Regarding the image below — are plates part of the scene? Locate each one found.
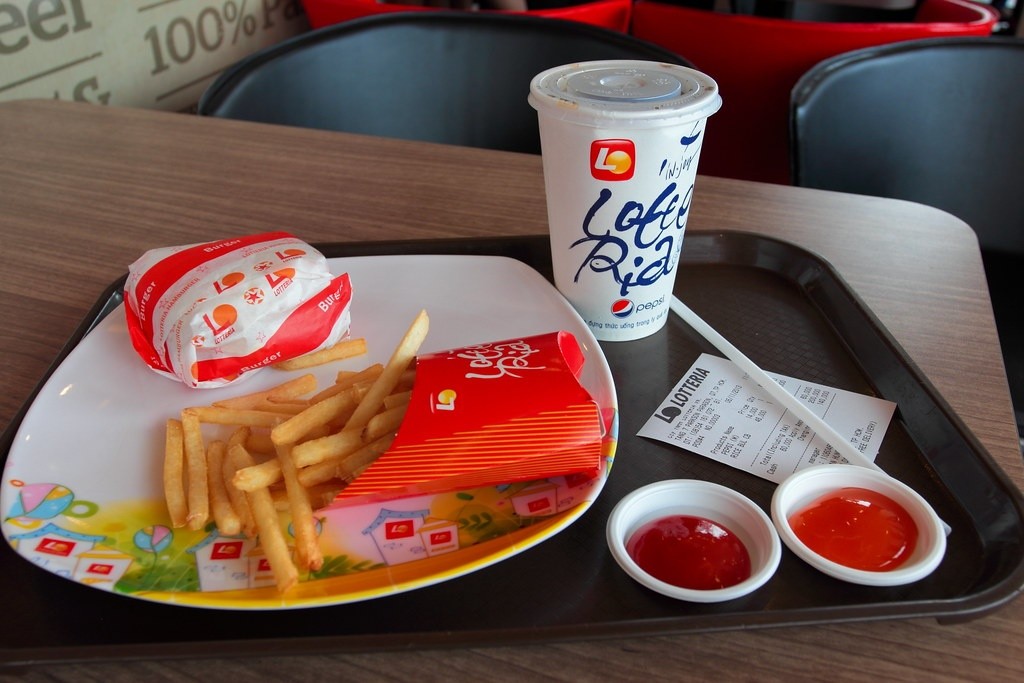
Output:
[0,255,619,609]
[769,463,948,586]
[606,477,781,602]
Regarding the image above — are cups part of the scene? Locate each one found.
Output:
[526,60,721,342]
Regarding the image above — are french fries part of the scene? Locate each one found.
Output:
[164,309,430,595]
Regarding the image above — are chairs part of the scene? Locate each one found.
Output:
[192,0,1024,255]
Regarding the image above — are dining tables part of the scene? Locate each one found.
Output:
[0,98,1024,683]
